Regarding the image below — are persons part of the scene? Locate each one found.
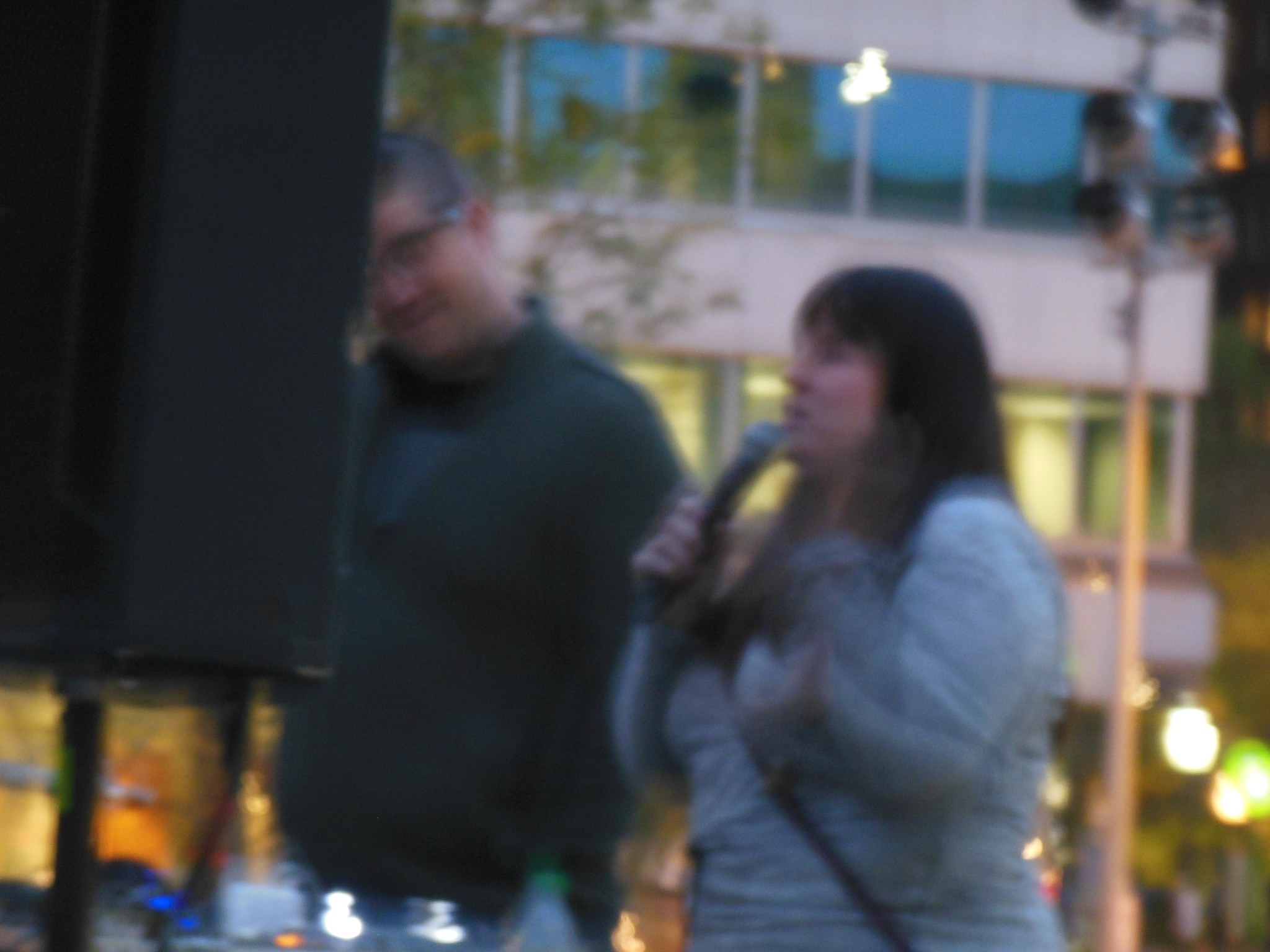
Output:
[271,132,696,952]
[609,266,1074,952]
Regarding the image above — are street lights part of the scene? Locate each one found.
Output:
[1062,1,1245,952]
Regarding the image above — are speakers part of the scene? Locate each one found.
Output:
[0,1,393,686]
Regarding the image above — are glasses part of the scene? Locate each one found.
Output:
[362,206,463,283]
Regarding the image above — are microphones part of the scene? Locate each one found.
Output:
[703,424,788,537]
